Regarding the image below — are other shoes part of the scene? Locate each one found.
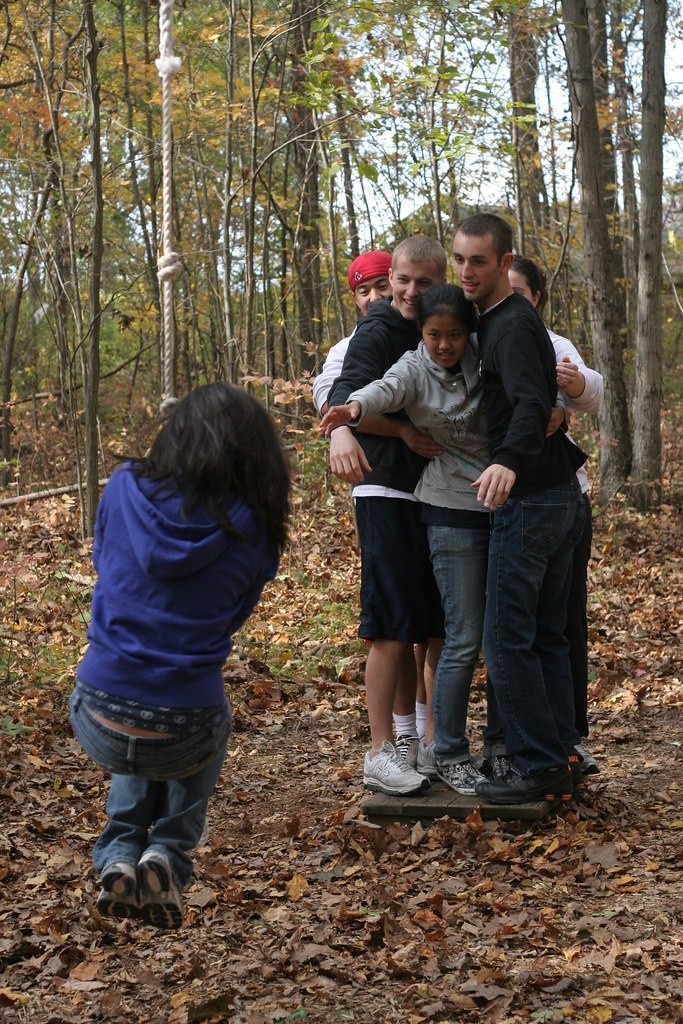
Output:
[136,852,186,930]
[97,863,140,918]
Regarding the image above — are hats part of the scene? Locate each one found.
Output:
[347,251,393,293]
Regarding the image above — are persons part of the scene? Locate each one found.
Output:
[313,213,604,806]
[69,382,291,930]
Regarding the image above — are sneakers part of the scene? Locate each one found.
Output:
[475,765,574,803]
[576,741,600,776]
[489,754,510,780]
[568,763,581,781]
[417,741,489,775]
[393,734,419,767]
[362,741,429,796]
[437,758,490,797]
